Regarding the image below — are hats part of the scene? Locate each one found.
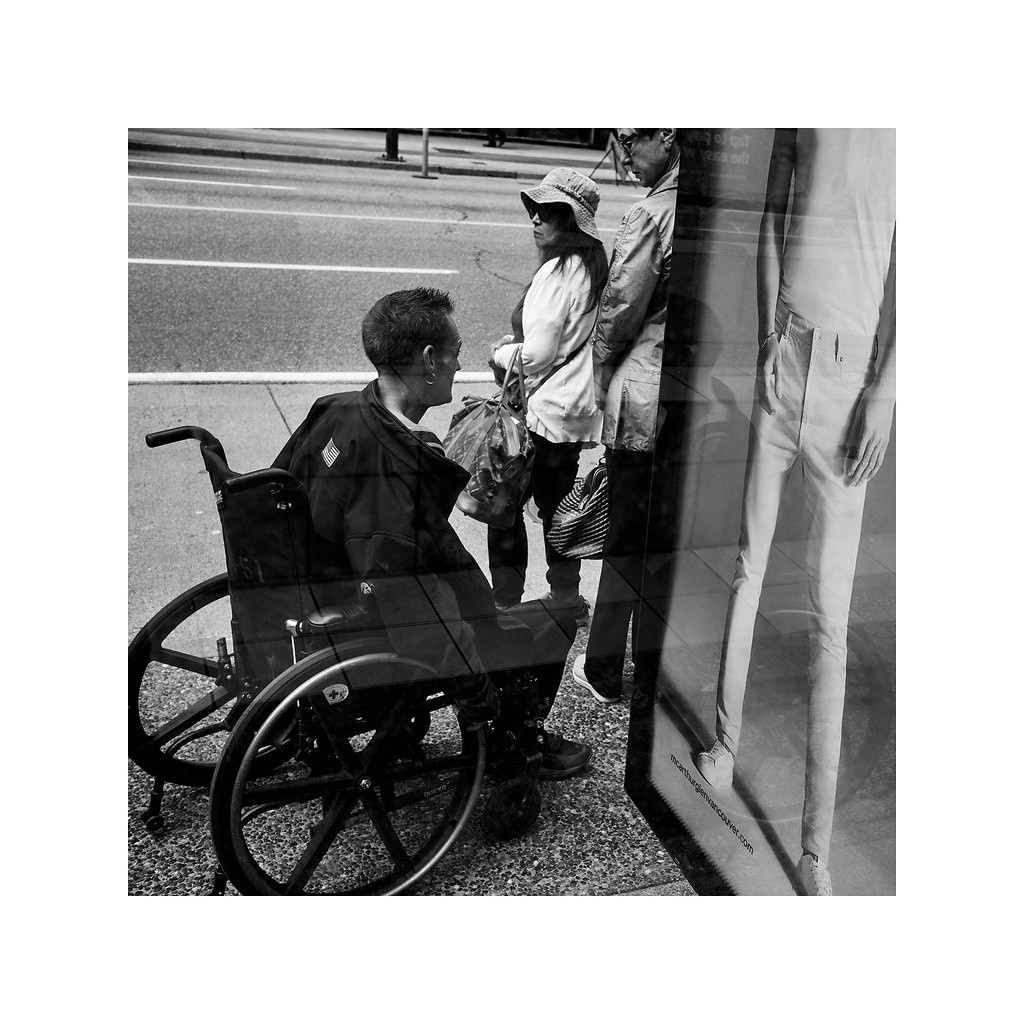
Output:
[519,168,601,241]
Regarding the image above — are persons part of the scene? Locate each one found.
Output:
[696,128,896,895]
[573,129,682,705]
[270,287,589,782]
[480,163,609,629]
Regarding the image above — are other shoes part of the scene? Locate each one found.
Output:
[483,717,592,778]
[483,135,506,147]
[542,591,589,626]
[572,653,619,703]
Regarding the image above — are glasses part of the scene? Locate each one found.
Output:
[618,133,636,152]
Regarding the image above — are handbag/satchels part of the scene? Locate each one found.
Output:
[443,344,535,531]
[546,456,610,560]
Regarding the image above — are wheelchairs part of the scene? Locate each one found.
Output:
[129,424,560,897]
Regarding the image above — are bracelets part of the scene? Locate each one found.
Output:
[758,330,777,353]
